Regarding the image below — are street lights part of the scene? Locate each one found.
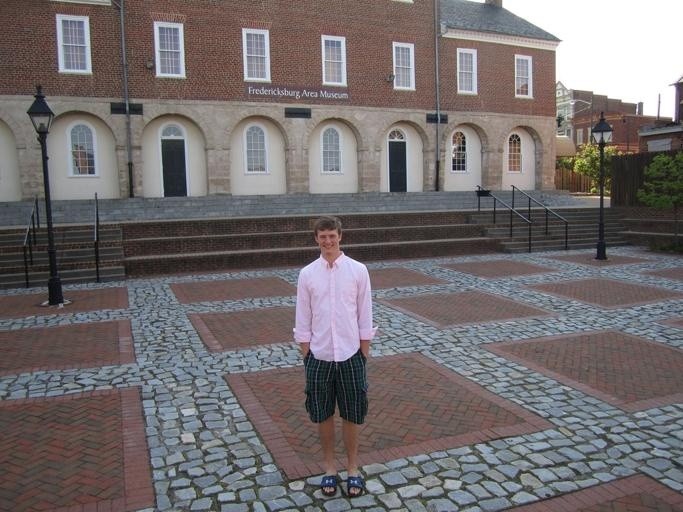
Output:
[569,97,594,146]
[24,82,65,308]
[591,109,616,261]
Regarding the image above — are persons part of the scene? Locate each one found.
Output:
[293,214,378,498]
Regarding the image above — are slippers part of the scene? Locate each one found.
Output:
[320,475,337,497]
[347,476,363,497]
